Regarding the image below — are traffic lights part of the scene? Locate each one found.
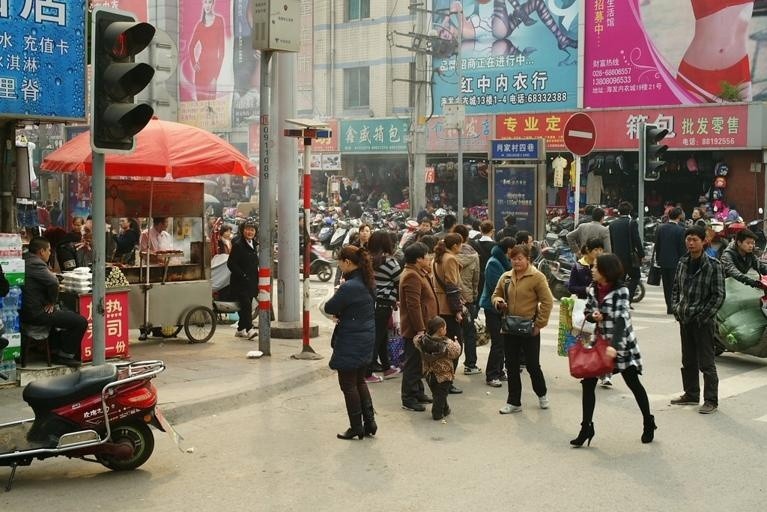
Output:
[94,10,155,150]
[645,125,668,179]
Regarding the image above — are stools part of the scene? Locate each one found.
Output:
[21,323,52,368]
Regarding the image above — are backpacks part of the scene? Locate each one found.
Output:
[593,153,698,175]
[436,162,488,178]
[712,162,729,213]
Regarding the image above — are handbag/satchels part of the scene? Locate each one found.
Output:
[648,266,661,285]
[110,249,135,265]
[459,324,490,346]
[387,336,401,367]
[375,297,395,316]
[567,337,616,378]
[571,299,597,333]
[500,314,534,337]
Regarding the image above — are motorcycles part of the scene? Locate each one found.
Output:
[0,327,184,492]
[220,200,457,283]
[713,254,767,358]
[538,204,767,304]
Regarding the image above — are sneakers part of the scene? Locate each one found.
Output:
[599,378,613,387]
[235,329,258,340]
[698,400,717,413]
[53,355,81,367]
[670,392,698,404]
[337,365,549,440]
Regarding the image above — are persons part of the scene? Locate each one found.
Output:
[570,252,657,446]
[187,0,227,100]
[669,225,726,413]
[227,221,258,340]
[432,0,579,56]
[324,203,553,440]
[720,229,767,288]
[578,205,595,226]
[451,38,536,63]
[609,202,645,309]
[675,0,754,103]
[339,178,410,218]
[216,224,232,254]
[568,237,613,386]
[654,201,708,314]
[0,213,176,355]
[566,207,612,254]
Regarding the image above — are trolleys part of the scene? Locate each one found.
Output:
[106,241,217,344]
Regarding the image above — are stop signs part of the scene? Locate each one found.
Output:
[564,113,597,156]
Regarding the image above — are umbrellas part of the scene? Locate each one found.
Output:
[40,116,259,327]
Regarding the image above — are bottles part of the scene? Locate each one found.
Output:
[0,232,23,260]
[18,201,39,226]
[0,358,17,383]
[0,286,23,335]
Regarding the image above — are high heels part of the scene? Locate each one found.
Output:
[641,414,657,443]
[570,422,594,447]
